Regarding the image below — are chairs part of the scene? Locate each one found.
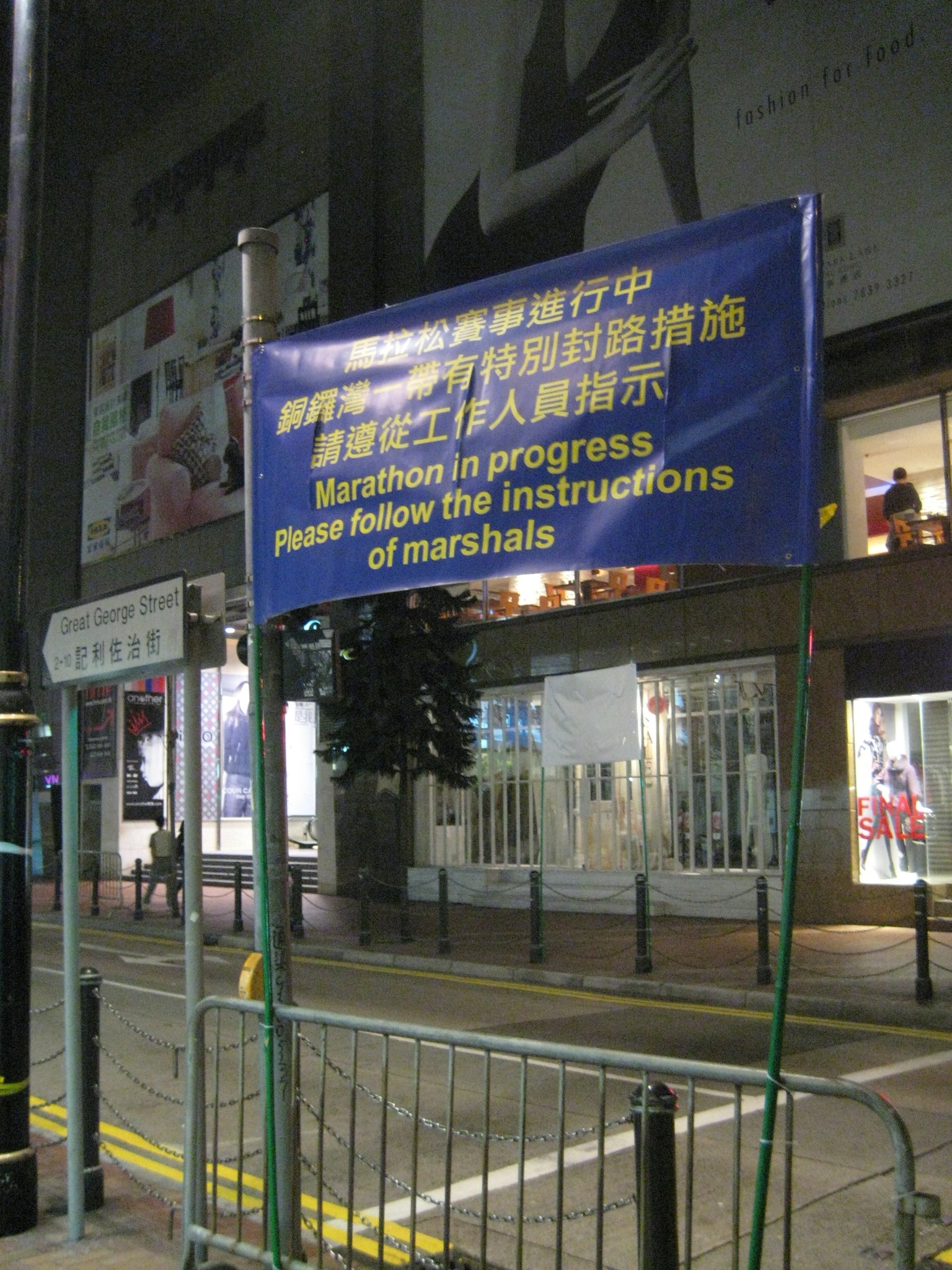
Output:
[892,517,919,548]
[466,570,667,620]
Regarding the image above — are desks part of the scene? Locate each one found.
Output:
[554,579,609,602]
[907,516,951,544]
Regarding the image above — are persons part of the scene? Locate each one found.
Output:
[424,0,703,299]
[882,467,922,552]
[857,703,897,878]
[127,709,164,800]
[141,816,184,918]
[873,741,932,873]
[221,681,252,817]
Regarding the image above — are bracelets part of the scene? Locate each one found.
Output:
[570,141,580,183]
